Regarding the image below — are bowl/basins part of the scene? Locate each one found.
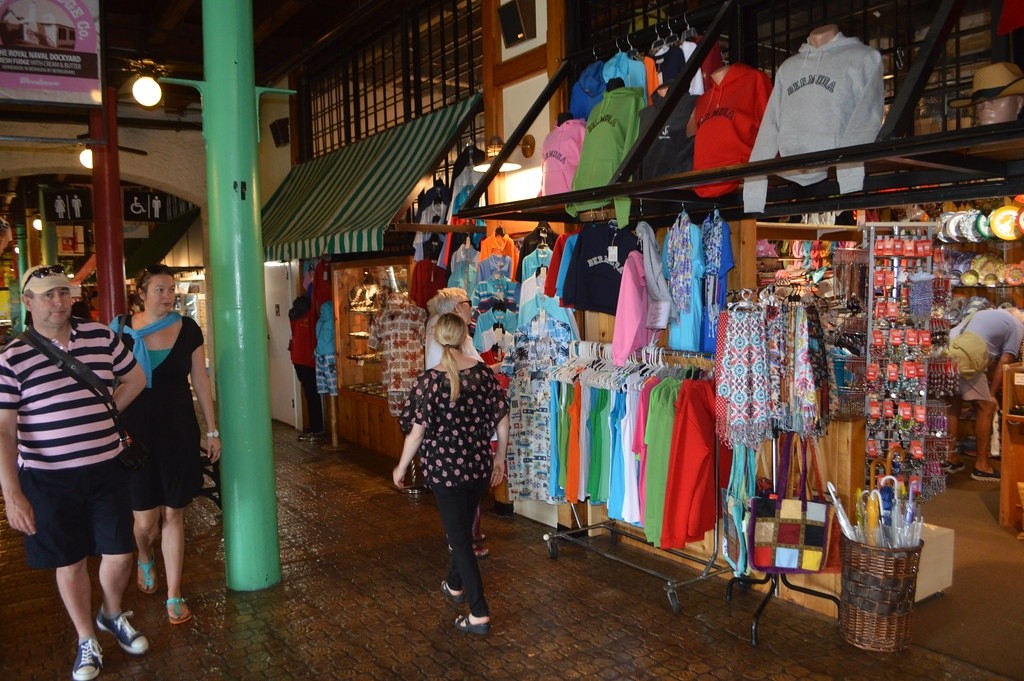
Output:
[984,273,997,286]
[961,270,979,286]
[946,270,962,284]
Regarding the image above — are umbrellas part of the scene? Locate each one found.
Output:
[827,446,924,547]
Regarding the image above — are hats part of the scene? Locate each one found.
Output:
[22,265,72,293]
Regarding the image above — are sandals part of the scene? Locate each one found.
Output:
[470,532,486,540]
[448,540,489,558]
[167,598,191,625]
[137,552,158,594]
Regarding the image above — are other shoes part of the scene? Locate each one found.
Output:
[945,461,964,474]
[971,467,1001,482]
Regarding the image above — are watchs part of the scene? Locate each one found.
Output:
[207,429,219,438]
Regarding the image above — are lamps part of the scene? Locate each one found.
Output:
[78,138,93,171]
[124,60,167,107]
[474,135,535,174]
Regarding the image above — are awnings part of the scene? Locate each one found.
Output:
[261,91,482,260]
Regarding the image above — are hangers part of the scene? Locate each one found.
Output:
[407,172,448,270]
[577,15,709,69]
[521,221,558,333]
[720,282,828,319]
[548,341,716,401]
[448,166,514,362]
[454,137,484,163]
[556,199,730,270]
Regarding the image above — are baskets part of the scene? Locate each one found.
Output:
[838,527,925,652]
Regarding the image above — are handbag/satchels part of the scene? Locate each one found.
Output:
[938,331,990,380]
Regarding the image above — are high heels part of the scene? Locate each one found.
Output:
[440,579,465,602]
[454,616,491,635]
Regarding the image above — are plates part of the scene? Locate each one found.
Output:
[936,206,1024,243]
[951,251,1024,286]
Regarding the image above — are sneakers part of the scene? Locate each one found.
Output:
[96,607,149,655]
[72,636,102,681]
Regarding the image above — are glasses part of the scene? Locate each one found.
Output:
[22,265,68,295]
[453,300,472,310]
[138,264,170,289]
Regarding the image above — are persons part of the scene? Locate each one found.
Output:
[392,312,510,632]
[425,287,490,557]
[693,63,773,208]
[368,292,428,416]
[289,295,323,441]
[0,264,148,681]
[949,62,1024,125]
[949,306,1024,482]
[542,78,646,228]
[742,23,884,215]
[72,301,92,318]
[108,263,221,624]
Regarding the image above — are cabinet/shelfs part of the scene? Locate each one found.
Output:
[947,240,1024,289]
[1000,361,1023,530]
[330,256,415,458]
